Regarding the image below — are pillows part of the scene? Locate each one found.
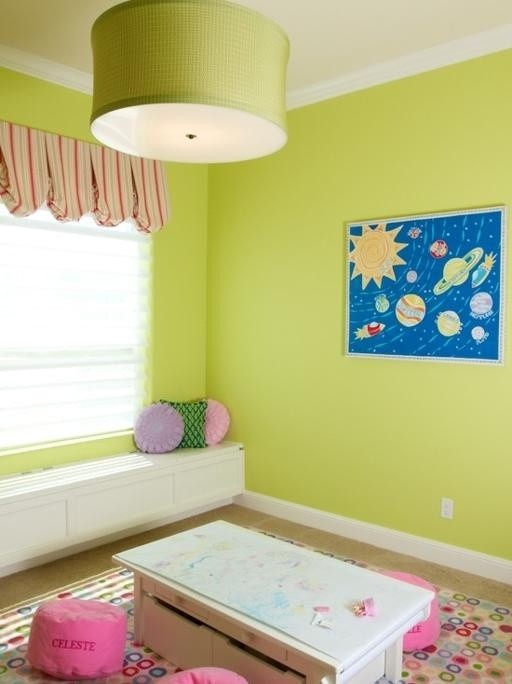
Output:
[134,403,185,454]
[159,399,209,447]
[207,399,232,447]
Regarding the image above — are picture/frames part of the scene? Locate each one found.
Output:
[341,205,505,366]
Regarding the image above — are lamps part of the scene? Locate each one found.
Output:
[90,1,290,165]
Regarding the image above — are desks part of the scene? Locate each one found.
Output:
[111,521,435,684]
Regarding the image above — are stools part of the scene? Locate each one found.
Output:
[384,572,440,652]
[25,599,128,679]
[153,667,247,684]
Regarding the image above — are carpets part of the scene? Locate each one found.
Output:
[0,528,512,684]
